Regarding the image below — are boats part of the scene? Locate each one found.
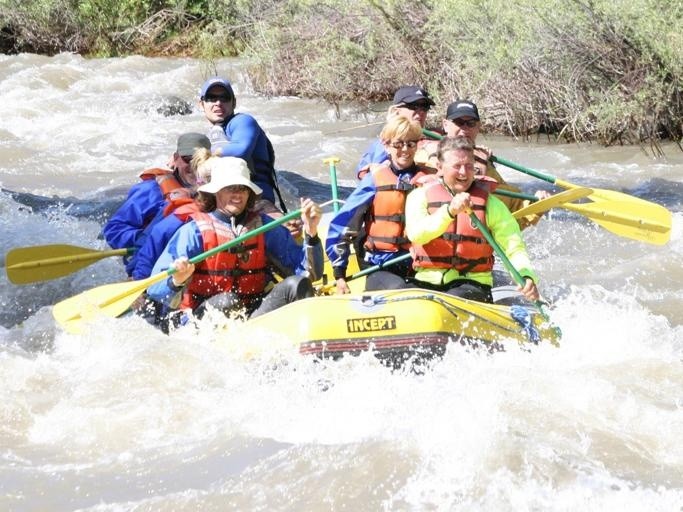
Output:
[238,211,562,371]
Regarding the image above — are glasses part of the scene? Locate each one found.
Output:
[180,156,191,163]
[452,118,475,126]
[392,140,416,149]
[226,185,247,192]
[204,94,230,102]
[401,103,429,111]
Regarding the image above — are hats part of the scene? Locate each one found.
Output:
[176,133,210,156]
[446,100,478,120]
[200,76,233,99]
[196,157,263,197]
[393,86,435,105]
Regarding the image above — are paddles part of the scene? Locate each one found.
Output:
[319,186,591,291]
[493,188,672,245]
[6,245,139,283]
[50,209,301,333]
[419,128,669,212]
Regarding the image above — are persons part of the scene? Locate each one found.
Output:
[144,155,324,331]
[196,76,279,203]
[357,85,492,184]
[406,135,539,307]
[415,100,551,234]
[100,129,301,311]
[326,117,432,298]
